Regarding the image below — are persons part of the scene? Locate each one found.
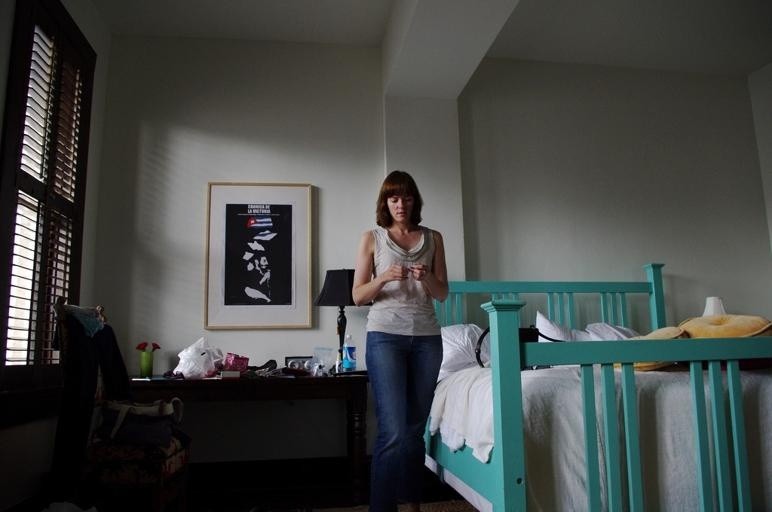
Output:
[352,170,449,512]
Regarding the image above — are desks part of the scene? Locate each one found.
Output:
[128,372,368,505]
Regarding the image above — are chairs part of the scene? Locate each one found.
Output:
[54,297,193,512]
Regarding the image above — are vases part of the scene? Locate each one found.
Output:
[141,352,154,378]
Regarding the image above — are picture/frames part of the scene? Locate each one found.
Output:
[285,356,314,373]
[203,181,313,329]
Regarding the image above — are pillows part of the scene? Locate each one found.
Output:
[678,313,772,366]
[436,323,491,383]
[535,309,640,367]
[611,325,690,371]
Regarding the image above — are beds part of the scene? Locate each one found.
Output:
[421,263,772,512]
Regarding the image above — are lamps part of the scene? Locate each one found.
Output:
[314,268,375,375]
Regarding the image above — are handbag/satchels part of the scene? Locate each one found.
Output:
[104,396,183,444]
[514,324,541,344]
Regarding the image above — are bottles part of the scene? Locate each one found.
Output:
[343,335,356,372]
[703,297,728,316]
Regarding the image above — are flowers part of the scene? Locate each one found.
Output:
[135,341,160,352]
